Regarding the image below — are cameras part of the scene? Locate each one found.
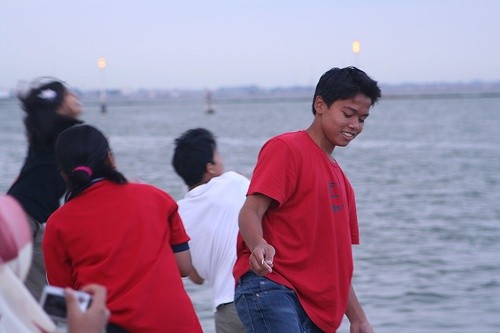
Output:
[40,285,93,324]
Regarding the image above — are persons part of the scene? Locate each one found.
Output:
[0,79,204,333]
[232,66,382,333]
[171,128,251,333]
